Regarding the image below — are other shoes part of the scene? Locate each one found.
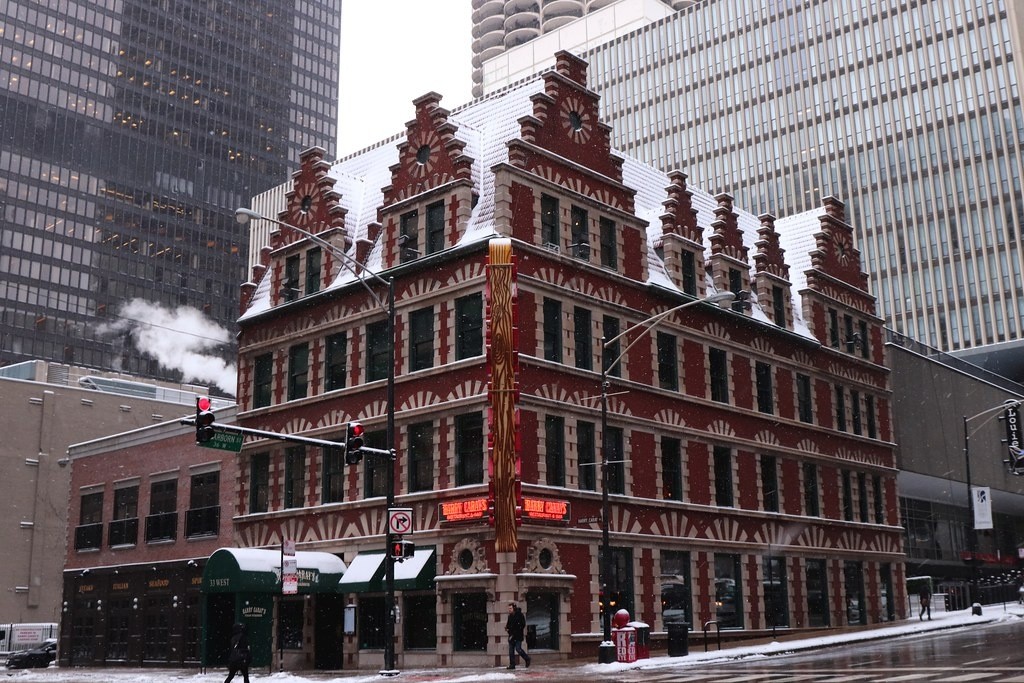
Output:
[507,664,516,670]
[919,614,922,621]
[928,616,931,620]
[525,657,531,668]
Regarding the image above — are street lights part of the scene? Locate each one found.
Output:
[594,288,737,669]
[233,208,401,681]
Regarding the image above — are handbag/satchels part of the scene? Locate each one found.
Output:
[228,633,250,668]
[921,599,930,607]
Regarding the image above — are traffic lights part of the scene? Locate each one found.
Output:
[195,396,215,443]
[343,421,364,467]
[390,540,414,558]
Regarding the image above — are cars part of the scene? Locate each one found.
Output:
[4,638,58,669]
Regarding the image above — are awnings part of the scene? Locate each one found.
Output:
[202,547,347,597]
[338,549,435,591]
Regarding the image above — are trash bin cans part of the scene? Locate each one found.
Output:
[667,621,690,657]
[598,640,616,665]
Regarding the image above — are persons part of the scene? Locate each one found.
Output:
[222,622,251,683]
[1019,583,1024,604]
[918,580,932,620]
[504,603,531,669]
